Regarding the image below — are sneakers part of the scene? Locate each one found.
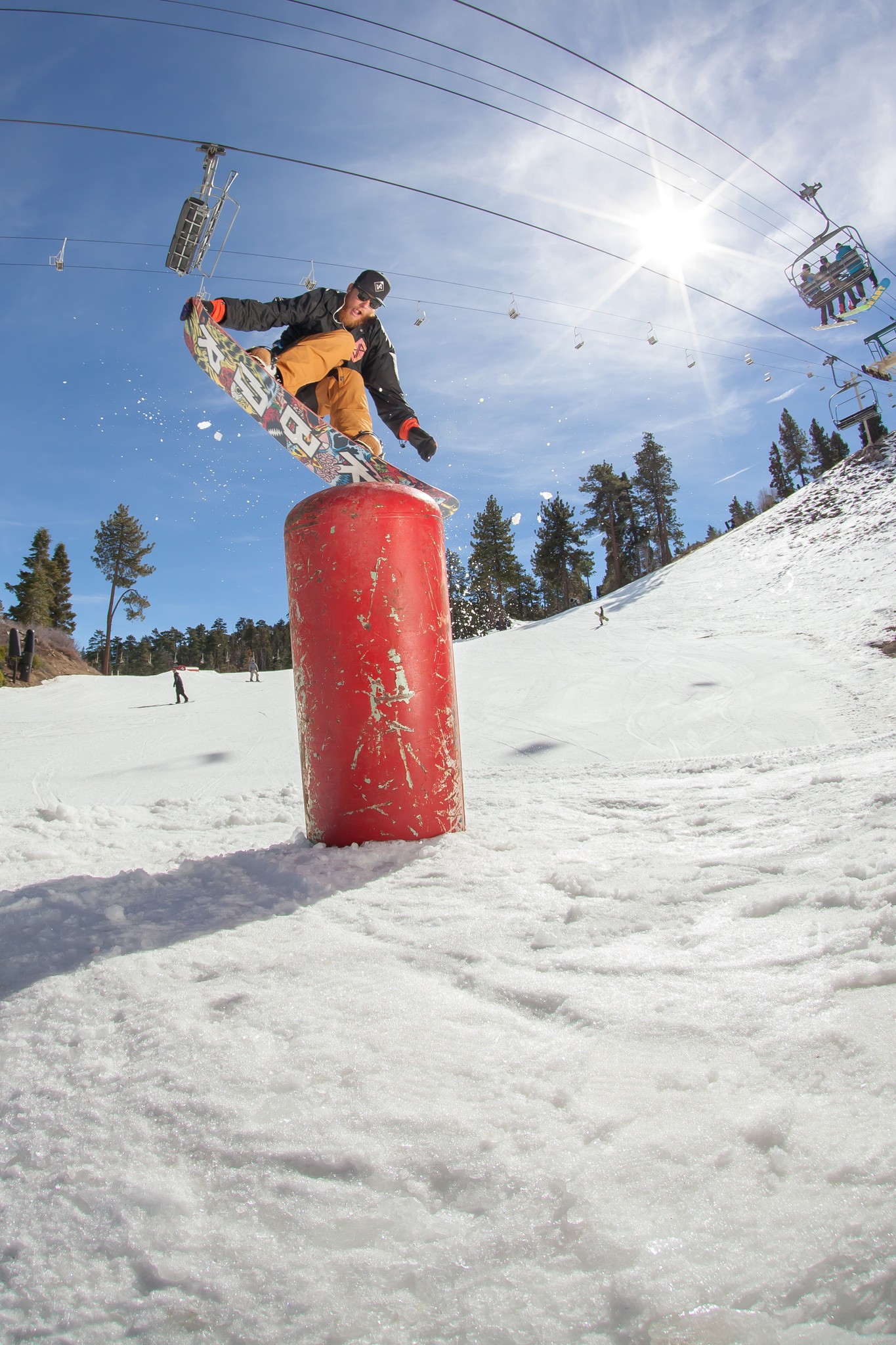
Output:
[820,280,880,326]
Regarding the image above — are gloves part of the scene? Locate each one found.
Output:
[180,297,226,323]
[398,418,437,463]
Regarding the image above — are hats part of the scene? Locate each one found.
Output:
[355,270,392,308]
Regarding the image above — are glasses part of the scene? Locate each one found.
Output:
[354,284,380,310]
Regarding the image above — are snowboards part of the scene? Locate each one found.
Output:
[595,611,609,622]
[836,305,868,319]
[860,278,890,311]
[245,680,264,682]
[169,700,195,705]
[811,319,858,331]
[184,296,461,521]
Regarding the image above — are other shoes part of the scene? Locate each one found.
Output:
[246,346,277,380]
[352,430,386,460]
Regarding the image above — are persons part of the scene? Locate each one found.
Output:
[172,668,188,704]
[800,264,838,326]
[179,269,437,463]
[820,256,861,315]
[599,606,603,625]
[249,660,260,682]
[835,243,879,303]
